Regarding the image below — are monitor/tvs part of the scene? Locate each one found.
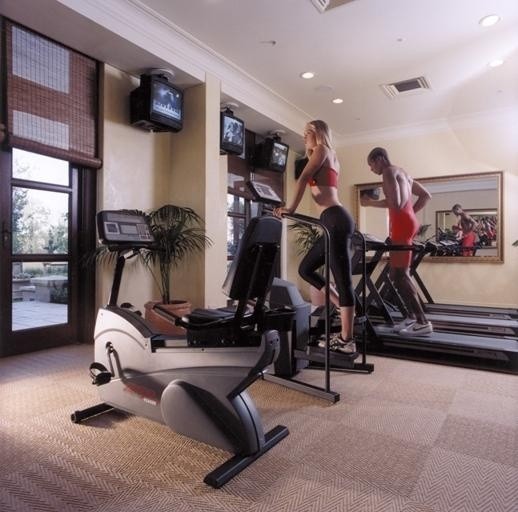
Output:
[360,188,381,200]
[295,157,309,179]
[130,75,183,132]
[220,111,244,155]
[256,140,289,173]
[247,180,282,205]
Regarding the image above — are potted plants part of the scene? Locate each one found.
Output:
[282,219,329,309]
[81,200,214,340]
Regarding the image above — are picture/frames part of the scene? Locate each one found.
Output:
[352,169,505,267]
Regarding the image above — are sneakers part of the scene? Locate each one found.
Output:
[399,320,433,338]
[393,317,417,334]
[318,333,357,354]
[335,307,357,320]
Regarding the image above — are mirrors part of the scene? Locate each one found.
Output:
[433,206,499,249]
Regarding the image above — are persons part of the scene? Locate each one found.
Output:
[272,121,358,355]
[358,146,434,338]
[167,92,180,112]
[447,202,499,256]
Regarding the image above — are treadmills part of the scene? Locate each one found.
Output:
[323,234,517,376]
